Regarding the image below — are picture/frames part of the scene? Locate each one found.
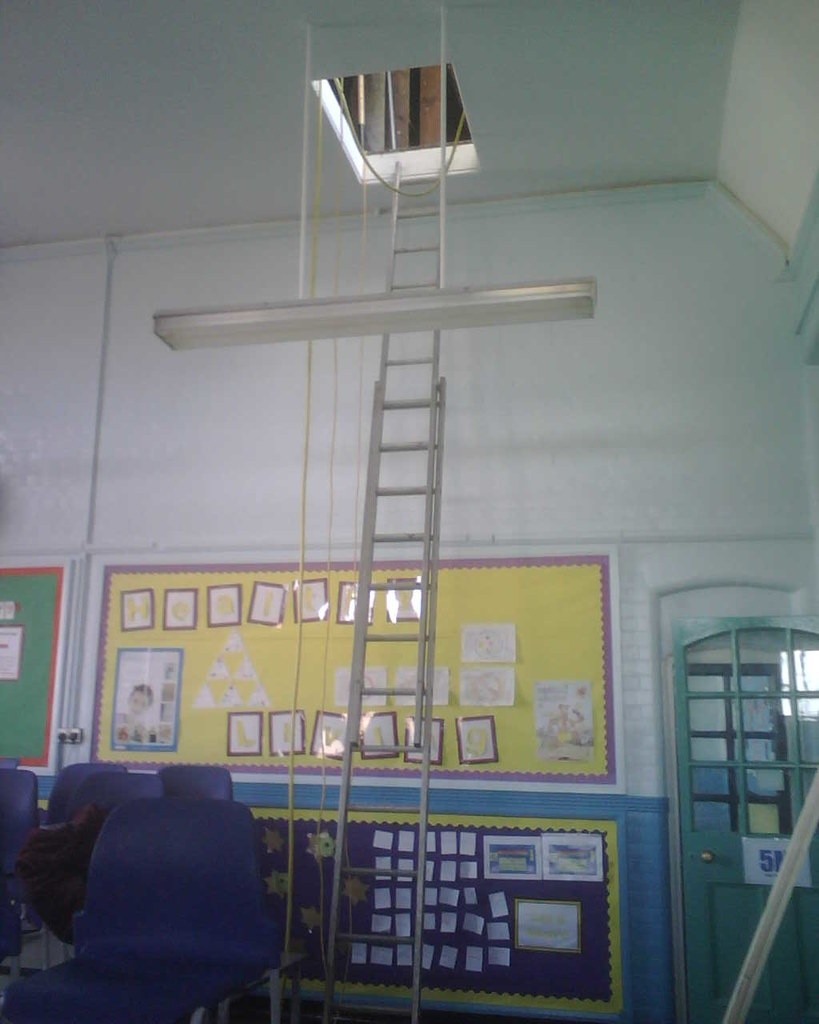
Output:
[514,898,582,955]
[119,588,155,632]
[109,646,184,752]
[292,577,329,624]
[246,580,288,629]
[335,581,375,625]
[207,583,242,628]
[226,711,263,757]
[162,588,198,631]
[0,624,26,682]
[357,711,400,759]
[309,709,348,762]
[267,709,306,757]
[386,575,422,623]
[403,716,444,766]
[455,715,498,765]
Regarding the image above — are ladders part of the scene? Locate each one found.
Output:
[320,148,447,1024]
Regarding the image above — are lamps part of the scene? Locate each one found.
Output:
[150,3,601,354]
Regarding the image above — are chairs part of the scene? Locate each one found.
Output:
[0,756,310,1024]
